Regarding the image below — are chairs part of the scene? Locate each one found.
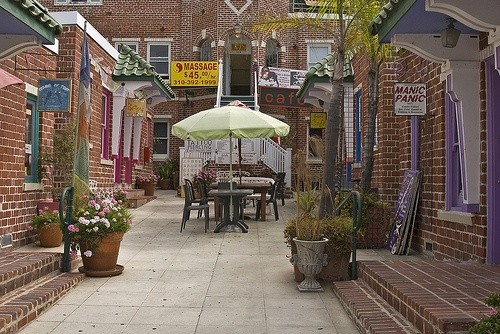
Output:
[180,172,286,233]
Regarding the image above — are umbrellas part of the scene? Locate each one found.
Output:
[171,102,290,233]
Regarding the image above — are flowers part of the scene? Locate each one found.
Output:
[26,206,67,236]
[137,171,160,183]
[67,188,136,258]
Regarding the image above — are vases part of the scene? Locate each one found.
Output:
[138,183,155,196]
[39,223,63,247]
[80,230,125,271]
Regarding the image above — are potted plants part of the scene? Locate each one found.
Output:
[283,135,393,292]
[159,160,178,189]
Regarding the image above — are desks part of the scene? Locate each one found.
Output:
[230,177,275,185]
[207,189,254,233]
[209,183,271,222]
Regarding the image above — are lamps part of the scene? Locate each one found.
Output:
[441,17,461,48]
[318,99,324,107]
[146,97,152,103]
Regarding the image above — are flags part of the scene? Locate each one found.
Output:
[71,20,92,216]
